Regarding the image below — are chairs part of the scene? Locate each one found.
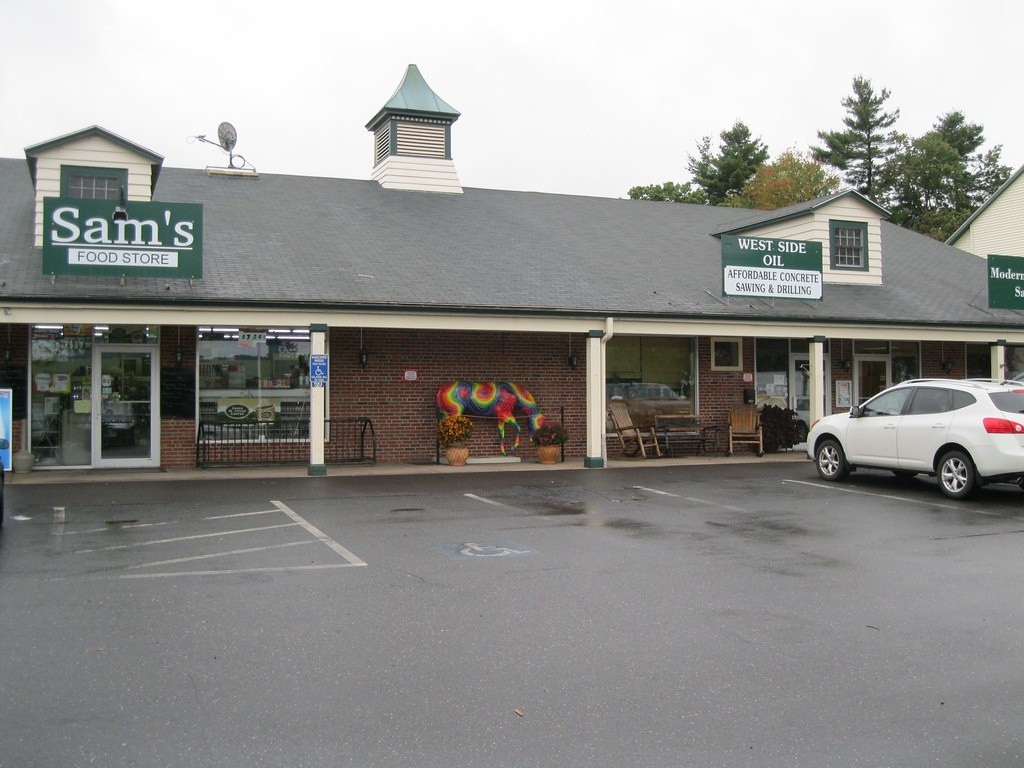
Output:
[726,405,763,456]
[608,397,663,462]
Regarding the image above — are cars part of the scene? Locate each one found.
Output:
[79,393,150,452]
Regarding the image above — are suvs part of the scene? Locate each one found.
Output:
[606,383,694,425]
[806,378,1024,501]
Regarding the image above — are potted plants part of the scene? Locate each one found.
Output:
[758,404,805,453]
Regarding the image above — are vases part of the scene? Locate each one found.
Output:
[537,445,561,464]
[446,444,468,466]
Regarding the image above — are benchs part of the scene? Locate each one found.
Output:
[653,414,721,458]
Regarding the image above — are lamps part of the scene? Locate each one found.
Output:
[942,355,953,374]
[840,355,852,373]
[111,184,128,222]
[359,347,368,368]
[568,349,578,370]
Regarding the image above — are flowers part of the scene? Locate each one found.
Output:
[530,424,568,448]
[438,415,474,447]
